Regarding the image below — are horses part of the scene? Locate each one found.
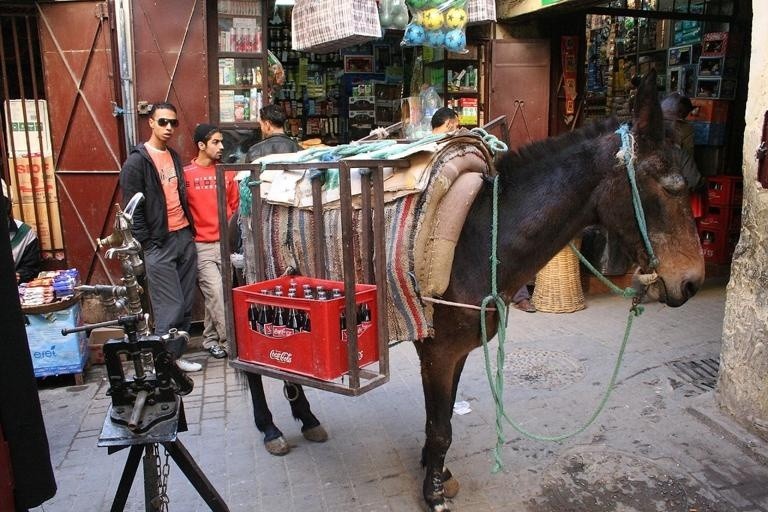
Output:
[226,68,704,512]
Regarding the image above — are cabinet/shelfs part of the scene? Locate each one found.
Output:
[423,31,482,134]
[208,2,271,131]
[271,1,422,146]
[584,12,670,126]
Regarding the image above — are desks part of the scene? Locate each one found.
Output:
[21,291,91,386]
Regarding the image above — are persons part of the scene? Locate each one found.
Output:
[120,101,205,373]
[657,139,705,192]
[245,104,306,163]
[660,93,695,157]
[0,177,44,287]
[430,107,461,134]
[182,123,240,360]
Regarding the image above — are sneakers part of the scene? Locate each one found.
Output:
[173,357,203,373]
[208,344,226,359]
[517,298,537,312]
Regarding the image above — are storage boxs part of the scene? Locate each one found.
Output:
[4,100,64,260]
[665,30,743,265]
[232,275,379,382]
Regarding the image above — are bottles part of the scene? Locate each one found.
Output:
[248,281,372,343]
[235,88,262,122]
[219,66,262,85]
[218,25,263,52]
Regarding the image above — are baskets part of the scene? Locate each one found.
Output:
[529,239,586,313]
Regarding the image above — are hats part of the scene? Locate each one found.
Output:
[192,123,221,145]
[661,94,700,119]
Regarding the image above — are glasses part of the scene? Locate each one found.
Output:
[154,118,180,129]
